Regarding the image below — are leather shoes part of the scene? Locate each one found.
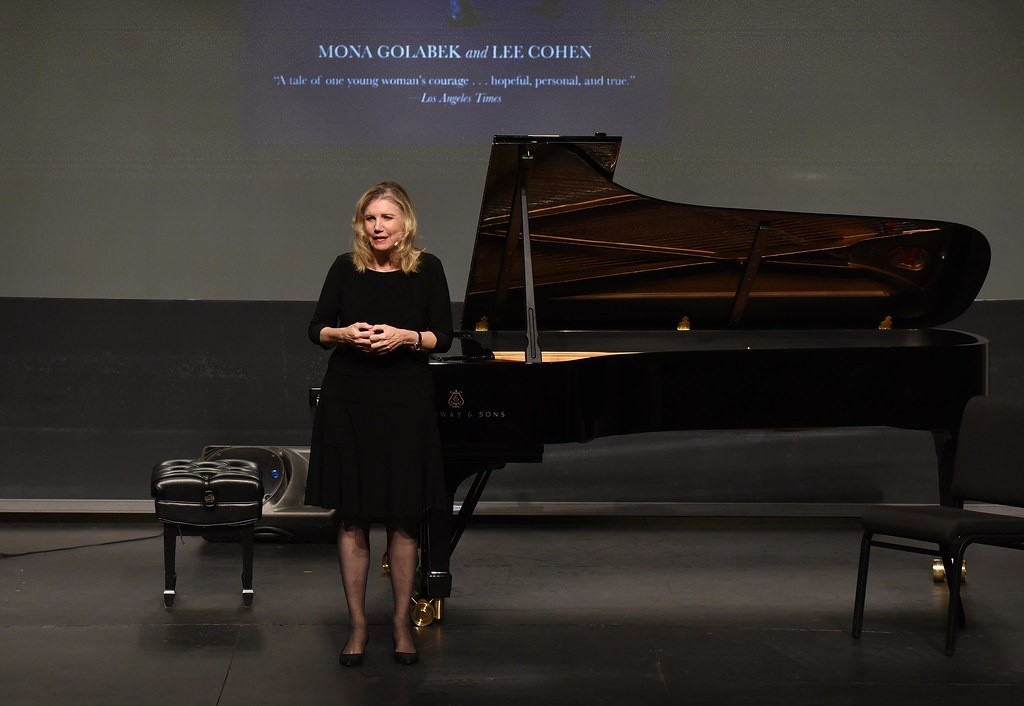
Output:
[339,634,370,667]
[390,634,419,665]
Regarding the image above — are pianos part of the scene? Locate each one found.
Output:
[377,130,995,629]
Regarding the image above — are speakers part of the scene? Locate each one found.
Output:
[203,445,334,539]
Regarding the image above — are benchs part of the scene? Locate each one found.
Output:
[150,459,265,607]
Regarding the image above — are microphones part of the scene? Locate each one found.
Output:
[394,239,401,246]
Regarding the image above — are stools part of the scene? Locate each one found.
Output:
[853,505,1024,658]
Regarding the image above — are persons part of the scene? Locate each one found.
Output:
[305,182,453,666]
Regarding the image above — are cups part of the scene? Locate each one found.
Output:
[462,331,492,359]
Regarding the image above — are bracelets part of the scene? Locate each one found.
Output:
[410,331,422,351]
